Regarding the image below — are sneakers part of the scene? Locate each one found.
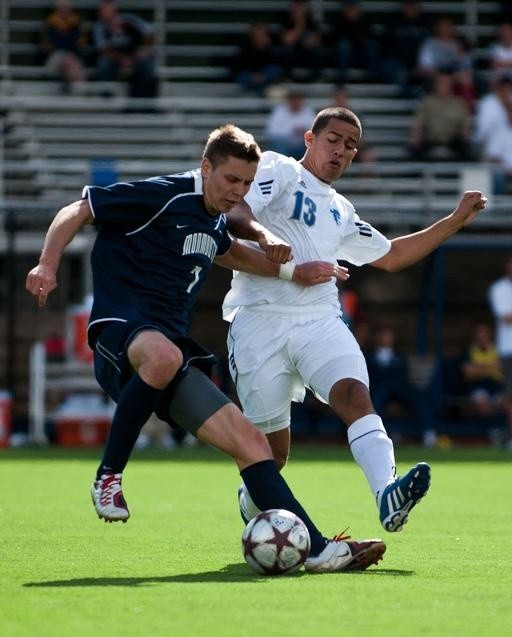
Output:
[375,461,431,532]
[238,487,255,524]
[91,472,129,523]
[304,537,386,572]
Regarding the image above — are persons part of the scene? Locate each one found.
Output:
[26,122,388,574]
[227,1,512,454]
[40,1,161,99]
[221,106,489,534]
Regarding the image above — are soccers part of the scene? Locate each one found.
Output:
[242,509,311,577]
